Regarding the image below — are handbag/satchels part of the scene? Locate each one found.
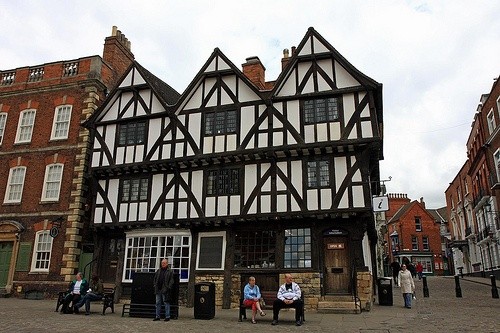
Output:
[260,299,265,307]
[412,291,416,300]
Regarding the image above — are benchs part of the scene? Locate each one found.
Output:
[122,304,179,320]
[239,289,305,325]
[55,288,116,315]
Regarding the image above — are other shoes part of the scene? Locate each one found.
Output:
[296,321,301,326]
[164,317,170,321]
[153,318,160,321]
[261,312,266,316]
[272,320,279,325]
[85,311,90,315]
[251,320,256,323]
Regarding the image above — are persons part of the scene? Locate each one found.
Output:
[390,259,401,287]
[75,273,105,315]
[271,273,302,326]
[416,261,423,281]
[398,264,416,309]
[243,277,266,324]
[60,272,89,314]
[152,259,174,321]
[408,264,417,279]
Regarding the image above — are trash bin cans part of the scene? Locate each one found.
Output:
[193,281,216,319]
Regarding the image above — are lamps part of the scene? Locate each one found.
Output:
[484,201,500,214]
